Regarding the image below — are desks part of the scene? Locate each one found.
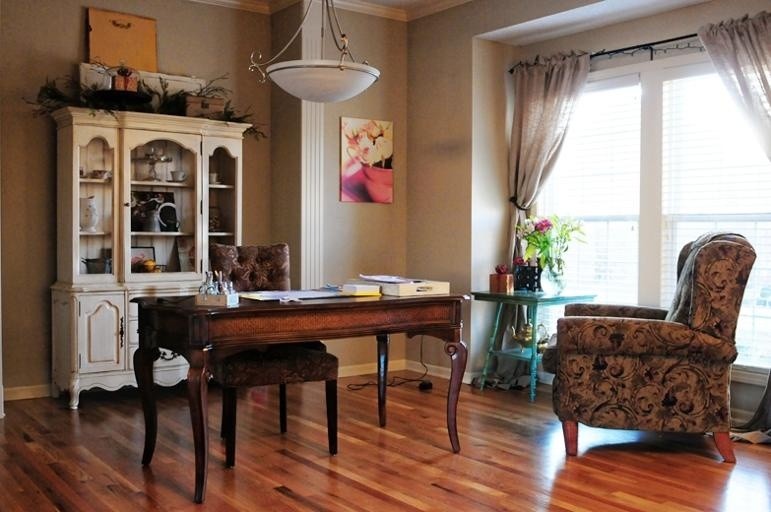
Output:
[128,286,471,503]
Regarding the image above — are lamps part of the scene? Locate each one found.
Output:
[246,0,382,103]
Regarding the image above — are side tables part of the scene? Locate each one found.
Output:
[470,287,600,402]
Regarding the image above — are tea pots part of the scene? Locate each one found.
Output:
[510,317,547,353]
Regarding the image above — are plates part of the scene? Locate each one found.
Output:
[165,179,186,184]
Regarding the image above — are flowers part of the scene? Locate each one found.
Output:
[515,211,587,294]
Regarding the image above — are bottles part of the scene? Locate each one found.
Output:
[80,195,99,233]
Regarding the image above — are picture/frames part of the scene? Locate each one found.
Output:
[131,245,155,264]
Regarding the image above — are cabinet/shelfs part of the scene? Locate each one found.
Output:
[50,106,250,412]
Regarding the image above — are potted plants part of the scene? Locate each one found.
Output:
[18,66,270,146]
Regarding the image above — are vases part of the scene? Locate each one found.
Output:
[517,255,544,292]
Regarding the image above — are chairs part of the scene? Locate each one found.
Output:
[206,238,339,469]
[544,230,762,473]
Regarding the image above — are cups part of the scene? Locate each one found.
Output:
[209,173,217,184]
[170,170,188,181]
